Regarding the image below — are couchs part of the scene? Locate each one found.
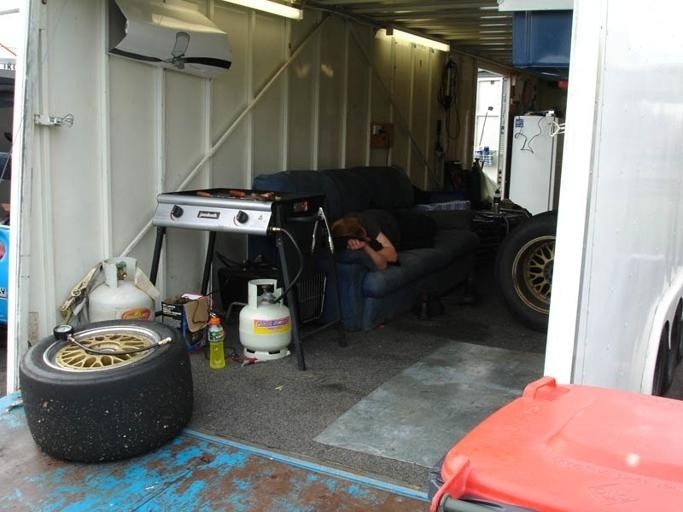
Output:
[250,165,479,331]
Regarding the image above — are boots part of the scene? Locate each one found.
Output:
[463,278,475,304]
[418,292,444,320]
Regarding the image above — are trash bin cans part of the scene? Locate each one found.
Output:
[429,377,683,512]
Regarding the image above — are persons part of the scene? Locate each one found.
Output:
[329,212,437,273]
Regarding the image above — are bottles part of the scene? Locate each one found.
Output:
[208,317,225,370]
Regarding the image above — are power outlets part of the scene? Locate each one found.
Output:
[373,125,384,135]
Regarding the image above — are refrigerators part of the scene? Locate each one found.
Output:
[508,115,563,216]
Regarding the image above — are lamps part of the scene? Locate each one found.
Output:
[374,29,450,53]
[223,0,304,21]
